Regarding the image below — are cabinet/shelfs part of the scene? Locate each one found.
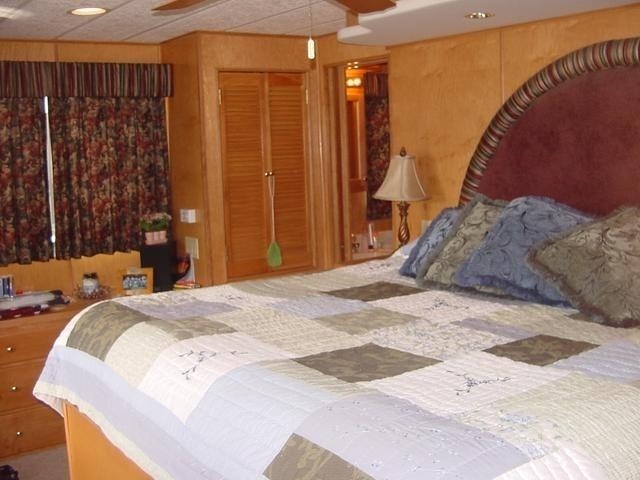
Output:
[166,70,314,279]
[0,299,107,462]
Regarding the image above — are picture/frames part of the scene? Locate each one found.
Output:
[115,265,155,296]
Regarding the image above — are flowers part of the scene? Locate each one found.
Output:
[139,211,172,232]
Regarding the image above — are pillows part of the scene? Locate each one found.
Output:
[399,194,640,328]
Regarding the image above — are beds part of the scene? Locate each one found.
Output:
[62,237,640,479]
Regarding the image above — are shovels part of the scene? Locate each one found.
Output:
[266,176,283,267]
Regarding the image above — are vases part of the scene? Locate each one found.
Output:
[143,229,167,244]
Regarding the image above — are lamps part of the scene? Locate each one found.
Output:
[372,146,429,246]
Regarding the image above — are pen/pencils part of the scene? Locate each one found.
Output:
[173,281,201,289]
